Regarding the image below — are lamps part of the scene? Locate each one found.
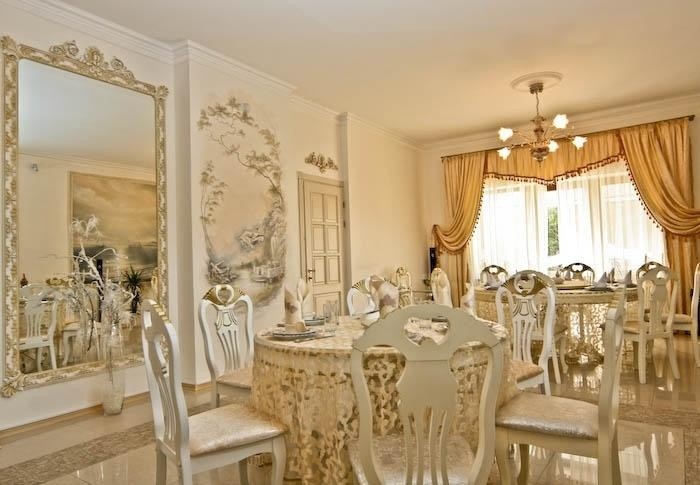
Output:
[495,74,590,164]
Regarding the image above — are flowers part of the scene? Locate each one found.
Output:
[47,215,132,393]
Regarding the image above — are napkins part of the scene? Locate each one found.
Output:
[282,280,309,323]
[376,284,403,320]
[458,282,477,315]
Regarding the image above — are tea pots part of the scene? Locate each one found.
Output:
[552,268,565,284]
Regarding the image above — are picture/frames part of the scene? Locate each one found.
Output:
[68,170,157,278]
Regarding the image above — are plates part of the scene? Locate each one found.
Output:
[614,283,638,288]
[486,287,499,291]
[588,287,613,291]
[269,333,315,341]
[605,281,619,284]
[277,314,327,327]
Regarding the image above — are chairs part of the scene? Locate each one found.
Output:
[391,267,421,308]
[428,268,454,309]
[347,276,386,315]
[18,272,137,375]
[494,308,627,482]
[138,302,291,483]
[494,273,558,398]
[252,316,513,461]
[200,284,258,407]
[346,305,504,485]
[473,264,699,385]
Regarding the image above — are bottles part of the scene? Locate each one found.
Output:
[20,272,28,288]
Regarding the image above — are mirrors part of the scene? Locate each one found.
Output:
[0,38,168,396]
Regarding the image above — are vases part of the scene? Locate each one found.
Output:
[97,325,126,414]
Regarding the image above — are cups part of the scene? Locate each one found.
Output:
[417,301,433,331]
[323,303,338,332]
